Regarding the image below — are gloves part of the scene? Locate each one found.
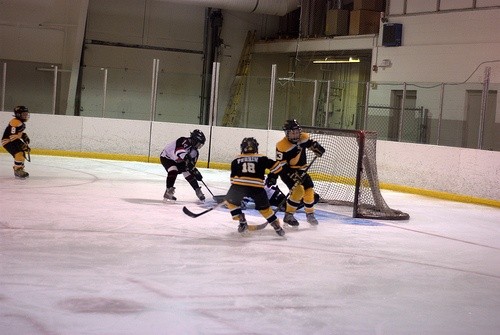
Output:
[287,169,303,184]
[189,168,203,182]
[266,172,278,187]
[183,154,194,169]
[308,142,325,157]
[22,133,29,144]
[20,143,30,152]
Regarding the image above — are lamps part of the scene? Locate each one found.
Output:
[314,57,361,64]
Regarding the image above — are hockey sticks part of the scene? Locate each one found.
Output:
[23,140,30,162]
[201,179,214,196]
[238,155,318,231]
[183,200,228,218]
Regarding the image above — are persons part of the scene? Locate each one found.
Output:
[224,119,325,237]
[160,129,207,201]
[2,106,31,179]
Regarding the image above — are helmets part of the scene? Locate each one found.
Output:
[14,105,29,122]
[191,129,206,149]
[282,120,301,144]
[241,138,258,155]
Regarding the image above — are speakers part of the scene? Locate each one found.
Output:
[382,23,402,46]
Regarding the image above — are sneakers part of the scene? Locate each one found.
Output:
[195,187,206,204]
[283,212,299,230]
[270,218,287,241]
[163,186,177,203]
[306,211,318,230]
[13,167,29,180]
[238,213,249,235]
